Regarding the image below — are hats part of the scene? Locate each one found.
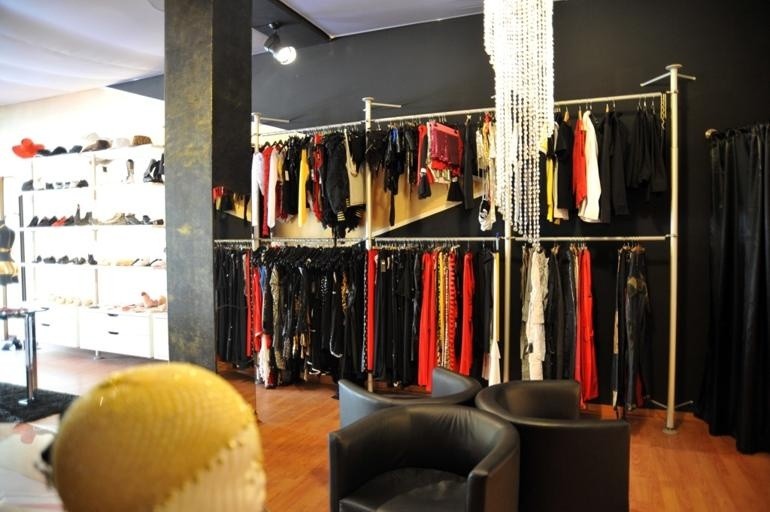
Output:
[11,133,151,158]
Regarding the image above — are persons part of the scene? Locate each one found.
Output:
[0,220,19,285]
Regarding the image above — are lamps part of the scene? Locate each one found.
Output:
[261,19,299,69]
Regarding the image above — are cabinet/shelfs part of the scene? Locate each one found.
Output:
[15,142,171,361]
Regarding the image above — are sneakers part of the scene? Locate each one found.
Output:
[37,179,89,190]
[50,295,92,306]
[121,293,166,312]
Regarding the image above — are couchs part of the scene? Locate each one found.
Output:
[472,373,633,509]
[337,365,482,431]
[326,399,525,512]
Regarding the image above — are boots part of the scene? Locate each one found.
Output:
[124,159,163,184]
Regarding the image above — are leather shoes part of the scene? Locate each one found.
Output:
[31,255,166,268]
[29,204,163,226]
